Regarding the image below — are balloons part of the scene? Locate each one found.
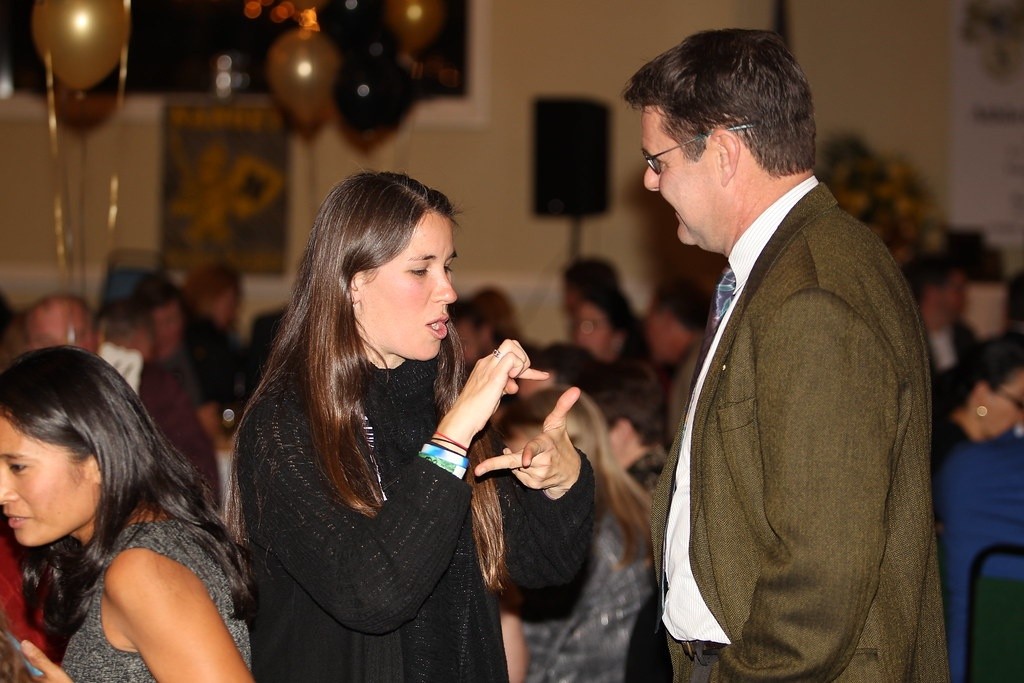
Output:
[268,28,340,136]
[329,49,415,153]
[32,0,125,93]
[390,0,441,52]
[316,0,387,55]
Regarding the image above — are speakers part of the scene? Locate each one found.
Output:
[531,96,610,214]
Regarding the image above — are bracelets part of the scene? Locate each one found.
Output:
[418,443,470,480]
[431,430,470,452]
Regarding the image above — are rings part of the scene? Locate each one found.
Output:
[492,349,502,357]
[517,467,521,471]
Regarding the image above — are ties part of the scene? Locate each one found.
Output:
[653,262,735,635]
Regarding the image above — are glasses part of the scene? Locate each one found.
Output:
[642,123,755,175]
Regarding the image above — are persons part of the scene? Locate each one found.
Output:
[0,346,258,683]
[622,28,953,683]
[441,253,716,683]
[223,170,593,683]
[900,222,1024,683]
[0,256,293,542]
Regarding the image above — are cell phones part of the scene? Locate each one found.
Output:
[4,631,44,676]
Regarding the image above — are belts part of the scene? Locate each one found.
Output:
[669,635,728,660]
